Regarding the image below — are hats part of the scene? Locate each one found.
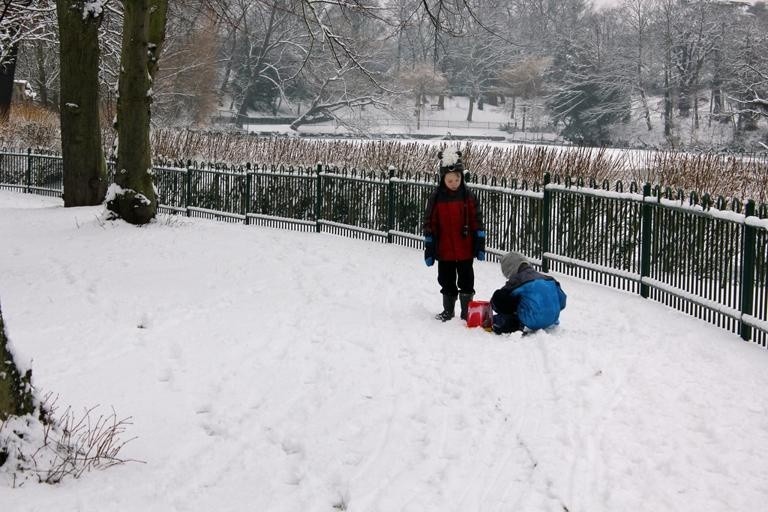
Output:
[437,151,464,187]
[500,252,531,279]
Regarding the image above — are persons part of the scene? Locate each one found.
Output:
[423,147,486,322]
[489,250,567,338]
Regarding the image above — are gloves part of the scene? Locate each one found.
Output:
[424,235,435,266]
[472,230,485,261]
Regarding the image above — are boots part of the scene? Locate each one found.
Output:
[435,288,474,322]
